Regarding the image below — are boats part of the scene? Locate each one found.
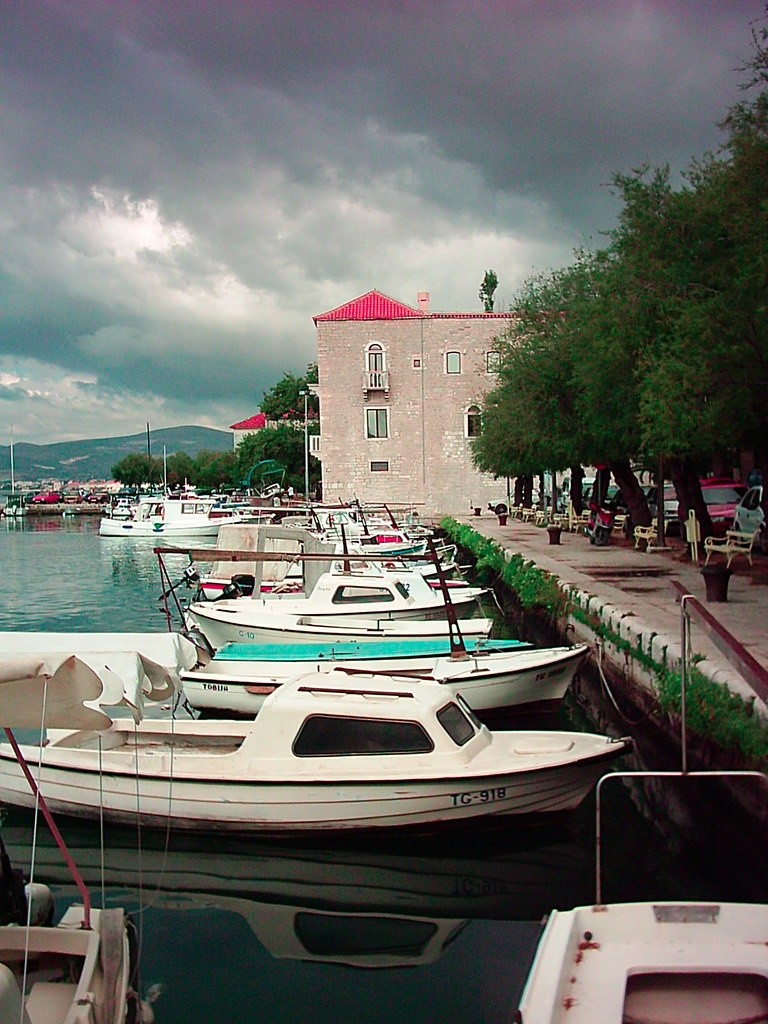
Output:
[0,676,630,832]
[1,494,29,517]
[186,523,489,634]
[177,535,588,715]
[98,497,470,598]
[511,772,768,1024]
[0,624,199,1024]
[0,811,667,973]
[191,601,497,652]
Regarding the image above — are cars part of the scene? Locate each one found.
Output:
[168,483,293,505]
[88,491,110,504]
[583,482,751,540]
[53,490,65,503]
[25,491,41,504]
[487,488,543,515]
[64,490,83,504]
[32,491,60,504]
[731,486,767,555]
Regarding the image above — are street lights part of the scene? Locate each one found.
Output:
[299,390,316,516]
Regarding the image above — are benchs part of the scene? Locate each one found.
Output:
[554,506,571,531]
[702,524,762,572]
[633,517,670,554]
[611,514,631,535]
[522,504,538,523]
[510,503,523,519]
[535,506,553,525]
[569,509,592,533]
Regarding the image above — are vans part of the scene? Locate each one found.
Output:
[562,477,597,514]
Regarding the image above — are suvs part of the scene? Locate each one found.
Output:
[113,487,136,504]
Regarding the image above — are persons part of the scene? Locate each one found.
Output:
[286,484,295,500]
